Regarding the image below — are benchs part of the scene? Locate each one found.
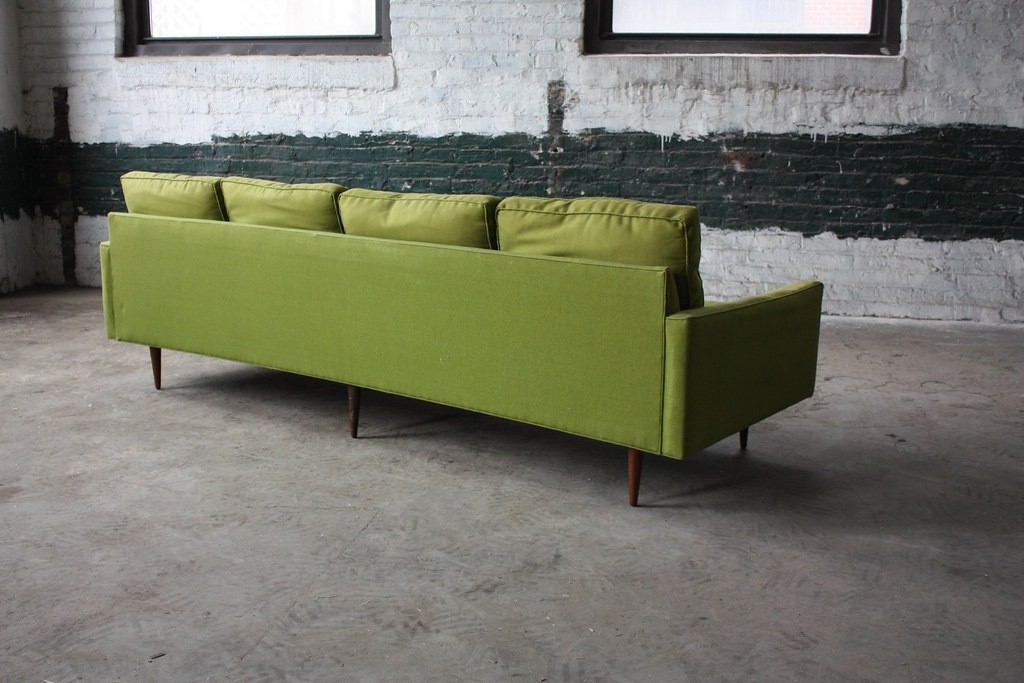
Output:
[100,171,825,506]
